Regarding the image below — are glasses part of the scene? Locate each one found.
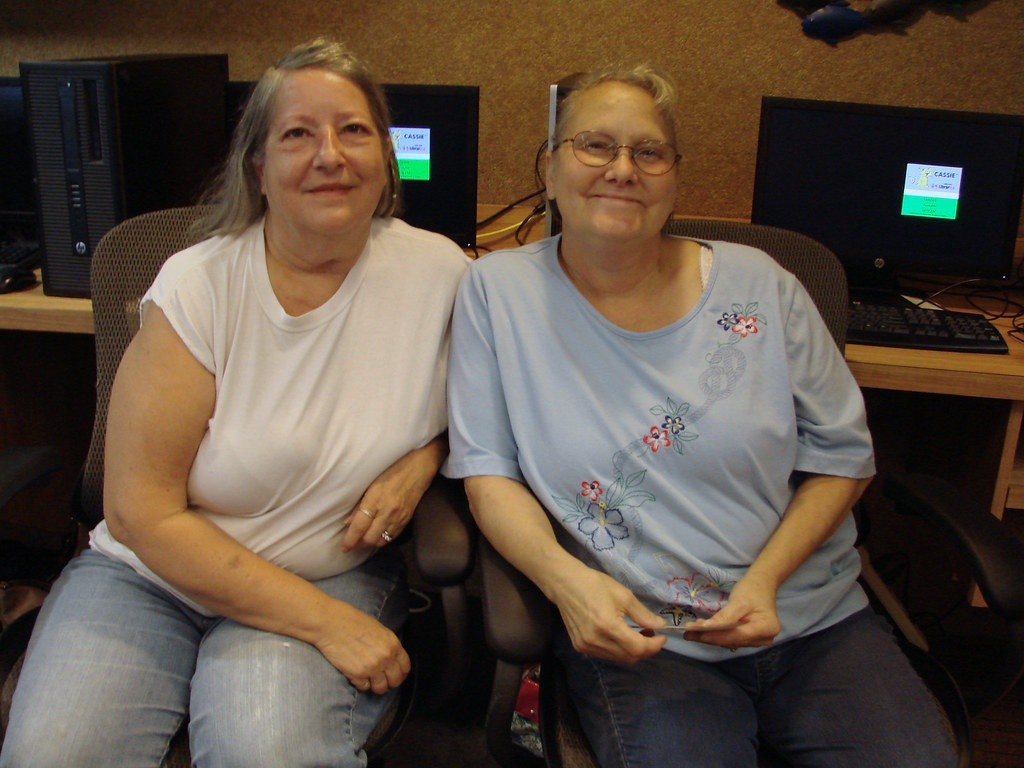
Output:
[552,130,682,177]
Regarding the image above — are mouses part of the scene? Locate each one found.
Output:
[0,269,37,293]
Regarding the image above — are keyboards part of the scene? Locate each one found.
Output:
[0,235,40,272]
[842,297,1009,354]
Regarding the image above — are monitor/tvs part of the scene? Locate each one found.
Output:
[223,79,479,248]
[0,76,39,240]
[752,97,1024,308]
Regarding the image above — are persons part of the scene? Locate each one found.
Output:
[0,37,474,768]
[443,61,955,767]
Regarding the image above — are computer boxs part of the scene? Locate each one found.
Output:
[19,52,232,298]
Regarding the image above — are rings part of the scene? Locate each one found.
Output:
[358,508,375,520]
[379,530,395,547]
[730,648,738,653]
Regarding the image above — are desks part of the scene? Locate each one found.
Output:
[2,259,1023,515]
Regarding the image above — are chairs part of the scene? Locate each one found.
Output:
[480,219,939,768]
[0,207,471,767]
